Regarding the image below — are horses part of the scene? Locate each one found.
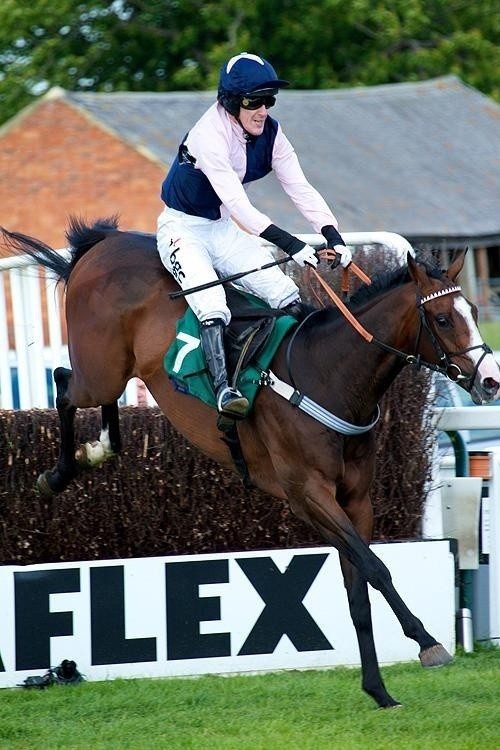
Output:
[1,212,500,709]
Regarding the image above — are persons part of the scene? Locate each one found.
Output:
[154,48,354,421]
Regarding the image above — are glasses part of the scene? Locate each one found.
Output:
[219,86,275,109]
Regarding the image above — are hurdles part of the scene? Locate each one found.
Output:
[0,357,464,690]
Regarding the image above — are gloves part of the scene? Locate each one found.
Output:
[327,245,353,270]
[292,244,320,269]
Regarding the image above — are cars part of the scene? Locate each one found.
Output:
[429,351,499,498]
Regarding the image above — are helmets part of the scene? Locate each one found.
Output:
[220,53,290,94]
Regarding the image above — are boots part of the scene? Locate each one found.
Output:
[199,319,248,432]
[282,297,302,315]
